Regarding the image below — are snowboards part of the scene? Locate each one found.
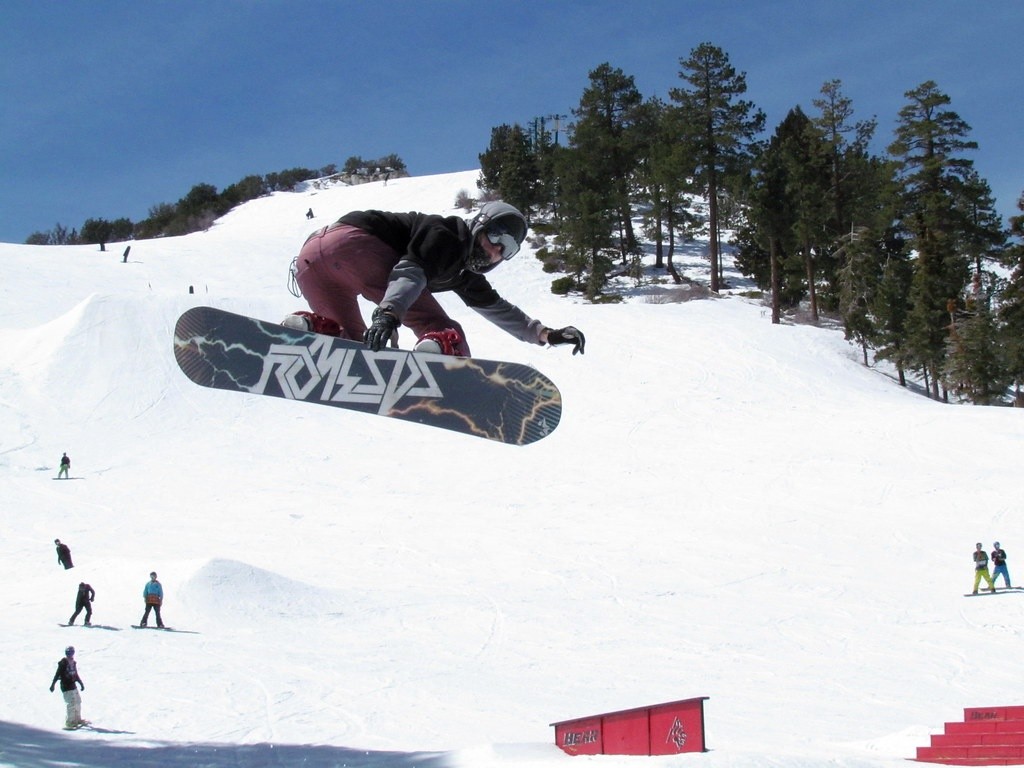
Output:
[131,624,171,629]
[62,721,89,730]
[52,477,73,480]
[964,587,1024,597]
[171,305,563,447]
[57,622,97,628]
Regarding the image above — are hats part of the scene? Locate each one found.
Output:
[498,216,526,246]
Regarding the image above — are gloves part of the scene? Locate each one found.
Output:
[547,327,585,355]
[363,307,399,353]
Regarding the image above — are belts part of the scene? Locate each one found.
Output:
[304,222,345,247]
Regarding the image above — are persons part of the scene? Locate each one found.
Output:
[68,583,95,625]
[140,572,164,628]
[280,202,586,358]
[58,452,70,478]
[49,646,85,727]
[55,539,73,569]
[973,543,996,594]
[990,542,1011,587]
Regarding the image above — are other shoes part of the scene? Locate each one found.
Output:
[283,315,311,331]
[414,340,443,355]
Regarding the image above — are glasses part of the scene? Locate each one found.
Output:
[487,227,521,260]
